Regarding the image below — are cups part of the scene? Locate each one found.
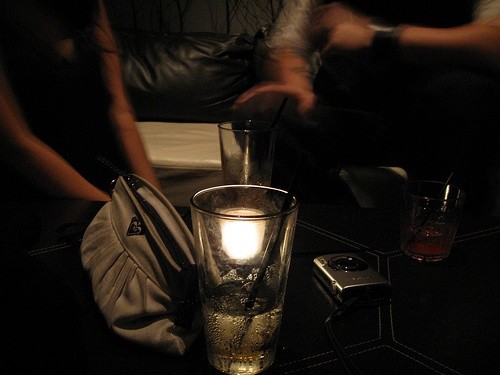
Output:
[191,184,299,375]
[218,120,279,190]
[398,181,466,262]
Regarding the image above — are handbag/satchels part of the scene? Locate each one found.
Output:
[81,173,204,354]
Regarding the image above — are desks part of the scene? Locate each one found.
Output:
[1,193,500,375]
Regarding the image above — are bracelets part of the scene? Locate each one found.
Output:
[369,25,403,53]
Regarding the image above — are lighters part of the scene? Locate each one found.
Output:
[313,252,391,303]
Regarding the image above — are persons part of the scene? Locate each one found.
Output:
[230,0,500,217]
[0,0,164,201]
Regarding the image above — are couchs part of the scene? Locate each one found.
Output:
[100,26,332,124]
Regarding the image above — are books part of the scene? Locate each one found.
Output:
[138,120,244,179]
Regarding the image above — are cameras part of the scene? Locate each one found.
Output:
[313,253,390,306]
